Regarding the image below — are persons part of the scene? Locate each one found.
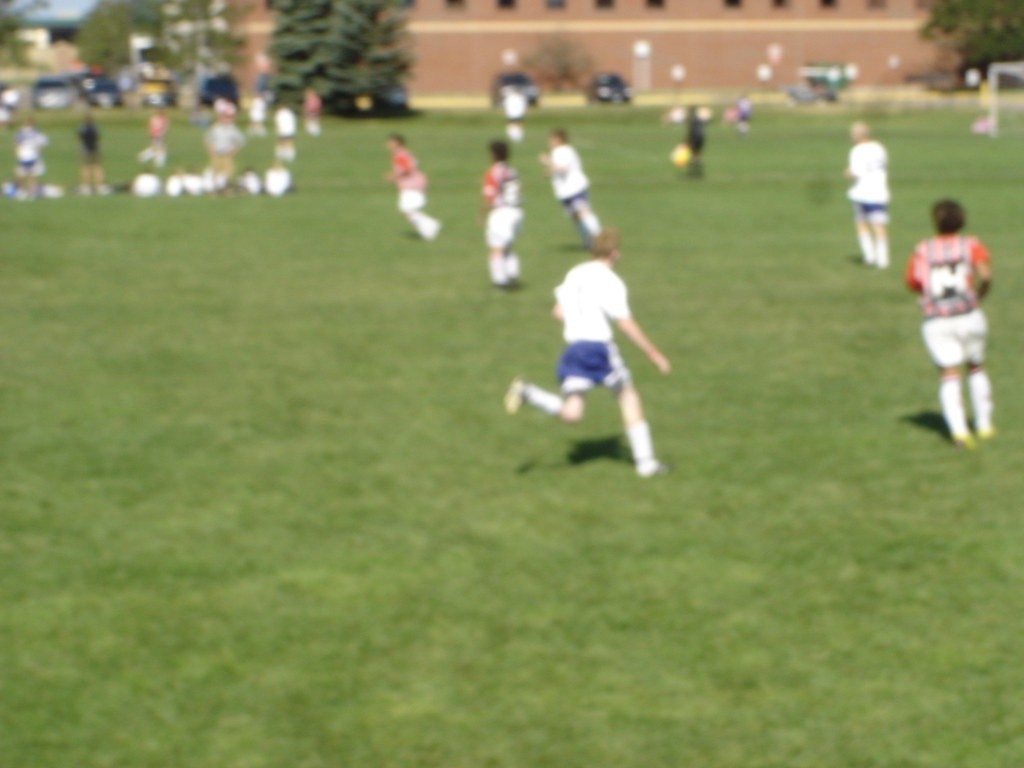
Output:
[386,132,439,236]
[540,129,601,248]
[505,230,670,475]
[0,82,319,201]
[488,49,538,140]
[685,105,706,176]
[482,139,522,284]
[845,121,891,268]
[907,200,997,448]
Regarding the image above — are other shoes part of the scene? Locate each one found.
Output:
[976,425,997,439]
[953,434,974,450]
[640,462,669,479]
[503,376,529,415]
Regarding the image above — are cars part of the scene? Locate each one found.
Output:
[583,72,633,105]
[199,66,275,107]
[490,72,540,108]
[325,80,408,116]
[26,64,178,114]
